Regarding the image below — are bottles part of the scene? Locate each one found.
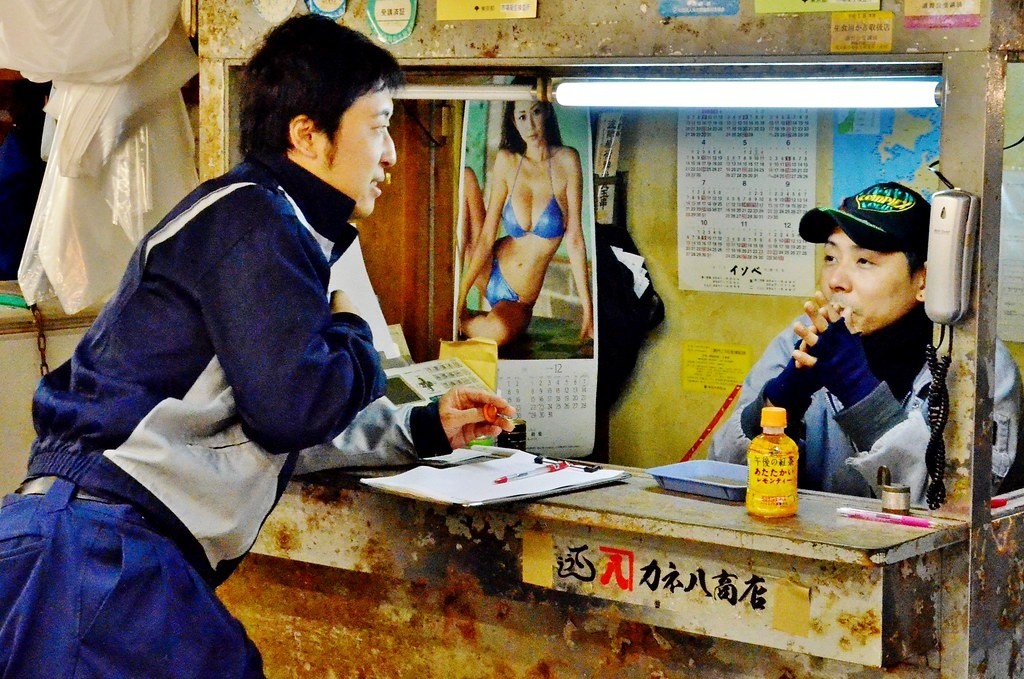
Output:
[746,406,799,518]
[469,435,493,447]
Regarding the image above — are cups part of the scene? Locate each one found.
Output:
[497,420,526,452]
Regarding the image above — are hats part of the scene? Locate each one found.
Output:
[798,182,931,254]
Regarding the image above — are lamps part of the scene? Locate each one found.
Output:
[387,75,944,109]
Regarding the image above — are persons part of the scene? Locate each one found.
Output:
[459,75,593,347]
[0,12,517,679]
[708,180,1022,508]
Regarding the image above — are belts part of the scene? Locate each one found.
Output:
[16,476,114,503]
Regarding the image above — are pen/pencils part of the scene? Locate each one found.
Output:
[835,506,936,528]
[495,462,568,483]
